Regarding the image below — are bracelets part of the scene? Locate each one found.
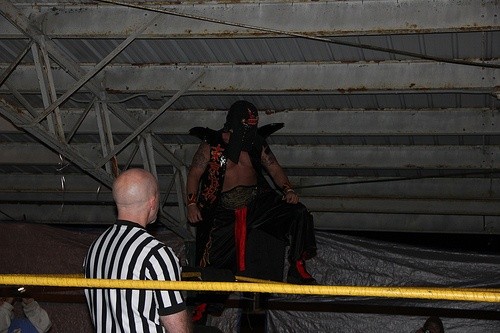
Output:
[286,189,294,192]
[187,193,197,207]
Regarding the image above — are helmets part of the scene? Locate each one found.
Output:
[224,99,259,150]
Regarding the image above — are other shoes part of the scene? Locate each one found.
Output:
[190,296,211,325]
[287,260,317,284]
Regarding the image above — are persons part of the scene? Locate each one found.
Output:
[83,168,222,333]
[186,99,317,326]
[416,315,444,333]
[0,298,52,333]
